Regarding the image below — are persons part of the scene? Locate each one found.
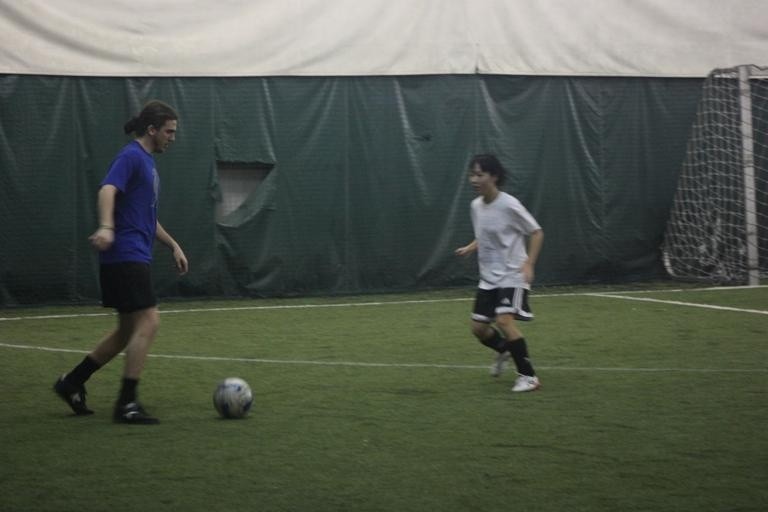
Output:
[53,99,189,423]
[454,153,545,392]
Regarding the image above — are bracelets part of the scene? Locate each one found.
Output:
[98,224,115,230]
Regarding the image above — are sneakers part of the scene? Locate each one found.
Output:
[489,351,510,375]
[512,376,539,392]
[54,374,94,415]
[113,403,159,424]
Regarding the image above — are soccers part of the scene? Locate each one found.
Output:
[214,378,253,417]
